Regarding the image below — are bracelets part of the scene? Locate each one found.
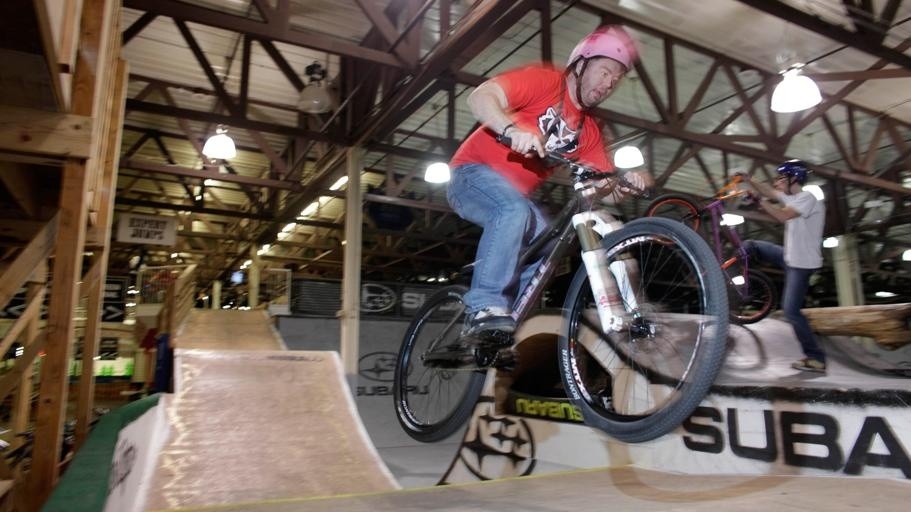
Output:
[753,194,762,203]
[496,122,516,142]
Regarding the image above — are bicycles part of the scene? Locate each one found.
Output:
[0,406,110,470]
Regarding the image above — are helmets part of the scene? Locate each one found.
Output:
[777,159,807,178]
[565,25,636,71]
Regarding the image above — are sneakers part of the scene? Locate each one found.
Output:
[793,357,826,374]
[460,308,517,342]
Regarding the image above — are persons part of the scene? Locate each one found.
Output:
[447,33,645,345]
[734,158,827,373]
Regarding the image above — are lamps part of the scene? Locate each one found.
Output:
[613,143,644,169]
[298,60,335,113]
[424,162,451,184]
[769,61,823,114]
[201,124,237,160]
